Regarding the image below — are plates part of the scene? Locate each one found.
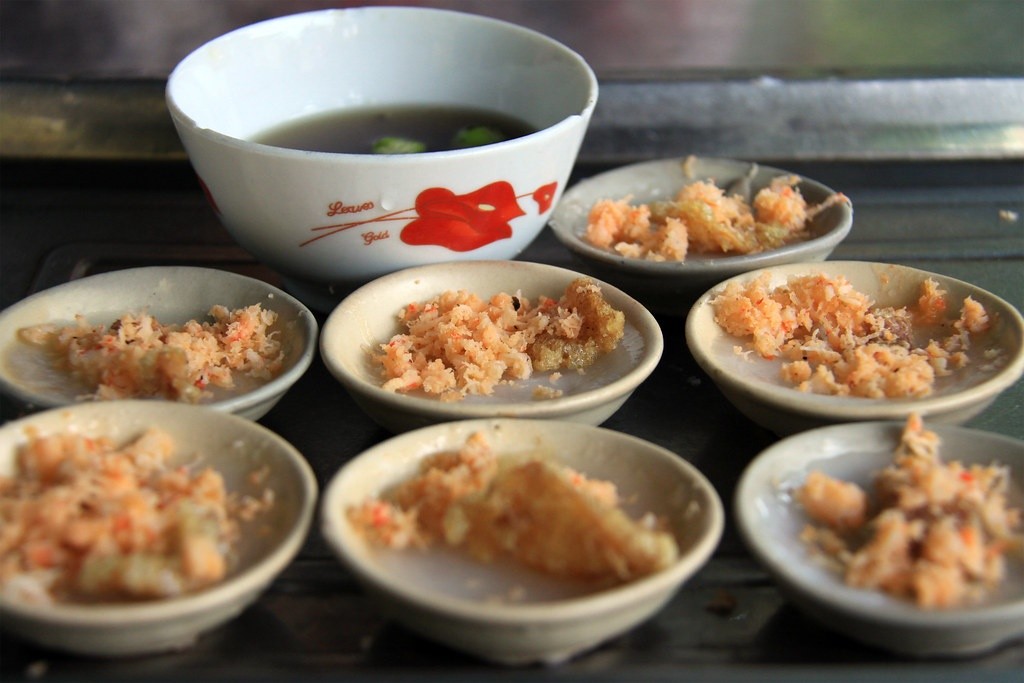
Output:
[547,157,854,315]
[736,422,1024,657]
[320,259,664,433]
[0,399,318,656]
[0,265,318,422]
[685,260,1024,439]
[321,418,724,665]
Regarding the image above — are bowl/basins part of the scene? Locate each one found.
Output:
[167,8,601,294]
